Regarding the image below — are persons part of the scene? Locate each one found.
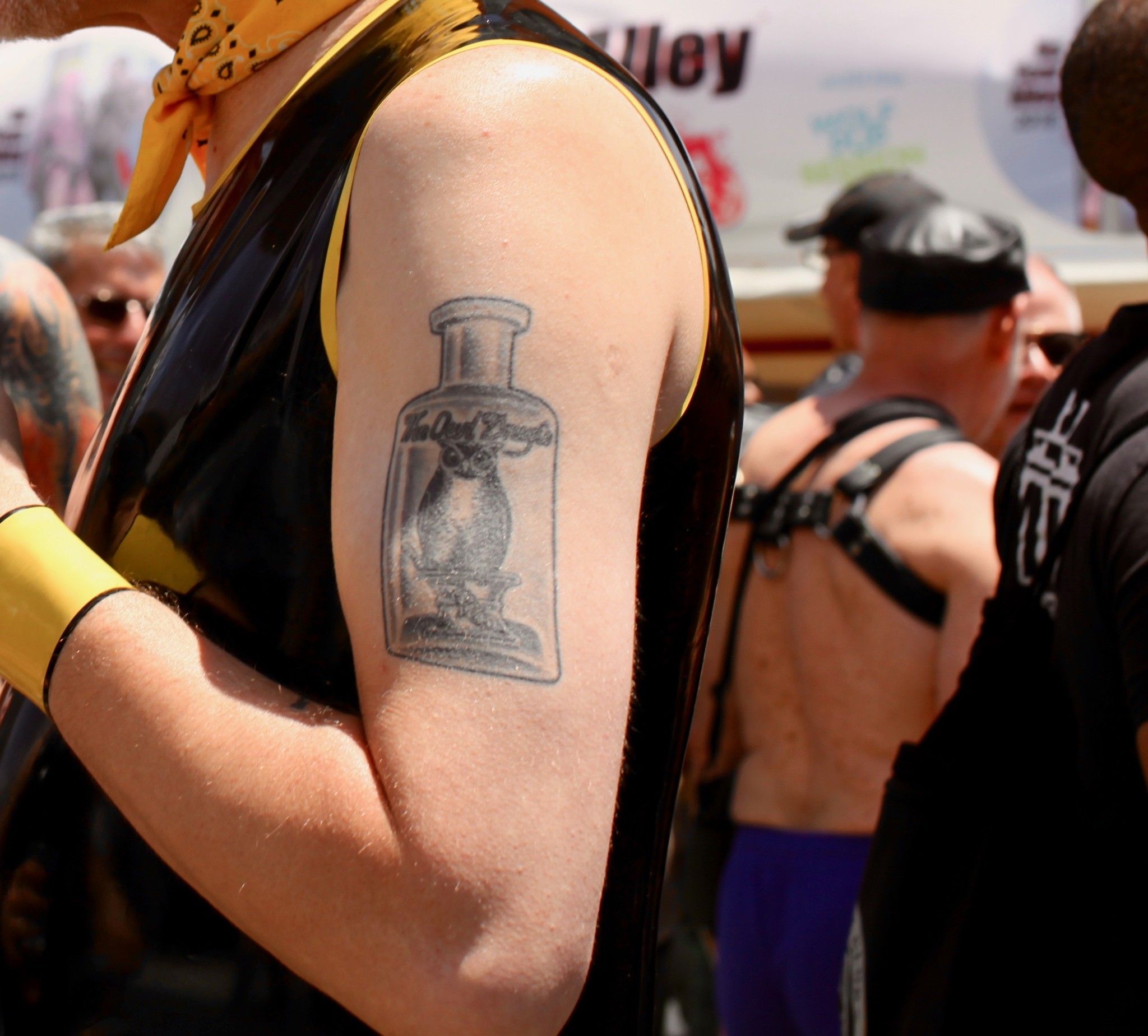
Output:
[0,0,1148,1036]
[0,0,744,1035]
[649,194,1031,1033]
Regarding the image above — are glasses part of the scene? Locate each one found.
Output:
[73,291,154,322]
[1037,333,1085,363]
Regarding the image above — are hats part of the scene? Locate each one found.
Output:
[856,202,1030,316]
[787,171,951,248]
